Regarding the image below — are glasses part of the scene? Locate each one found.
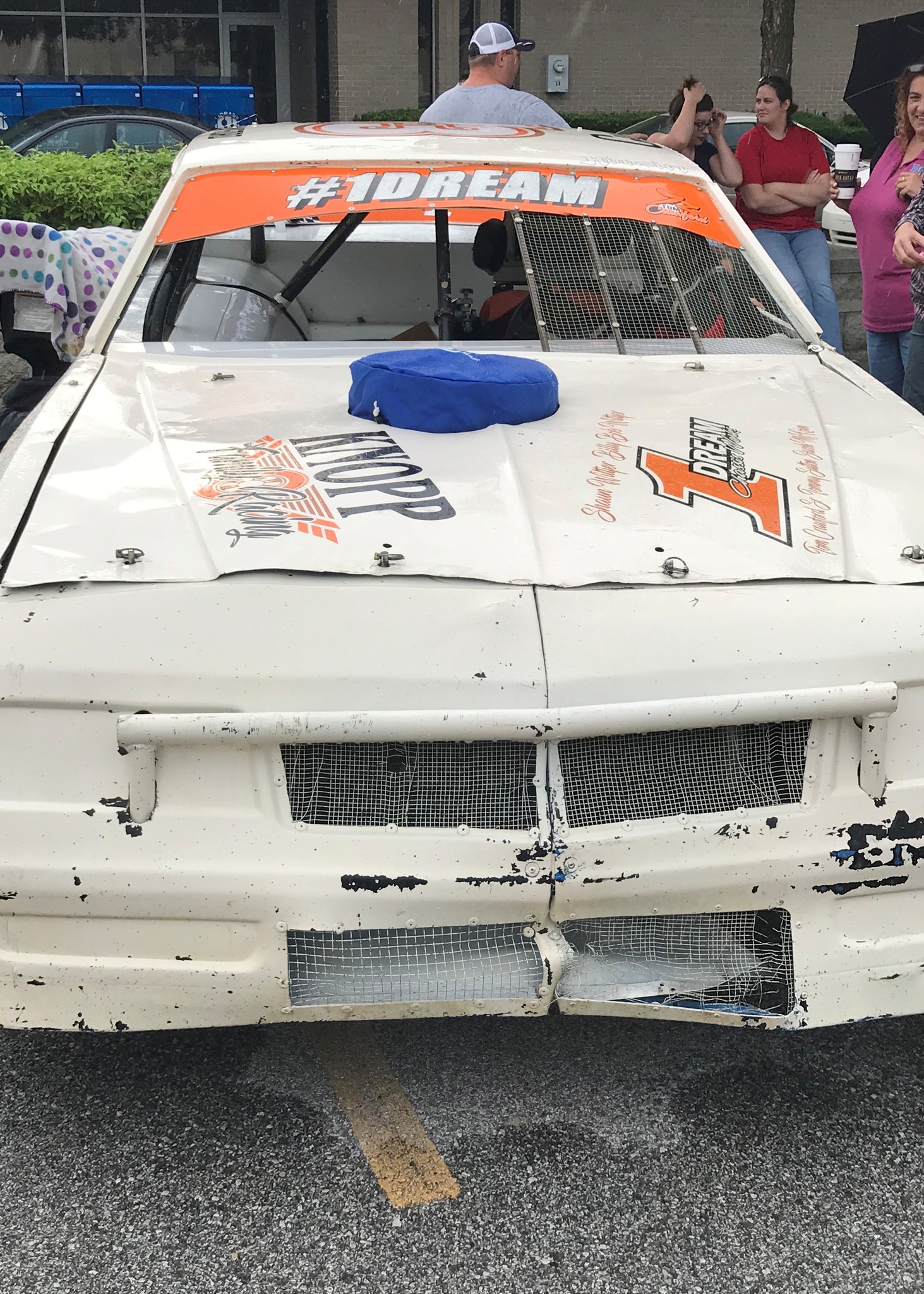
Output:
[693,117,715,131]
[908,65,923,72]
[759,76,786,89]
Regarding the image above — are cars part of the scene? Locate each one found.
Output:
[1,118,924,1037]
[614,110,838,212]
[0,105,214,160]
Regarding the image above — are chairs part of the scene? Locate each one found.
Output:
[473,210,688,339]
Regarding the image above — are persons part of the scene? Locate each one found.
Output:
[644,64,924,415]
[417,23,572,128]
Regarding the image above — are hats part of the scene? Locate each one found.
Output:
[468,22,538,56]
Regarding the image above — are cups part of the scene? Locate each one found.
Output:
[833,144,862,199]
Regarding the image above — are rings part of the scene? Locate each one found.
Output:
[902,176,905,182]
